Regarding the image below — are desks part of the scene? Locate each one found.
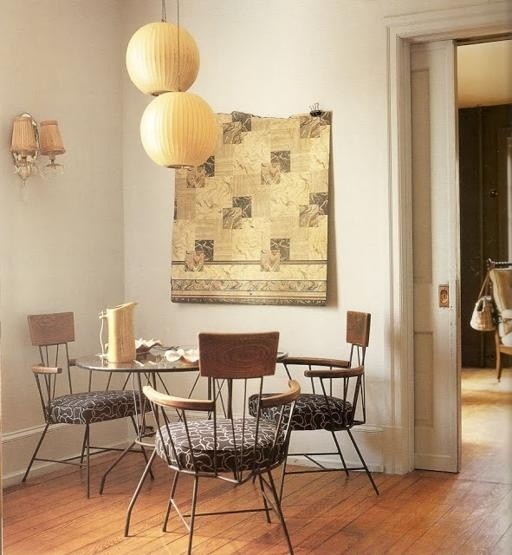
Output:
[73,342,290,496]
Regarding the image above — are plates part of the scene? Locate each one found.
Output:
[136,338,163,355]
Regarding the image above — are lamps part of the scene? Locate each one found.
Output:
[126,1,220,174]
[9,112,65,183]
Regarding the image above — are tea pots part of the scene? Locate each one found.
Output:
[98,301,138,362]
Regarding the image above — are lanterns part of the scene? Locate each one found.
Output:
[126,17,219,170]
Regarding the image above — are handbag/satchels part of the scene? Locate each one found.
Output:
[469,271,498,333]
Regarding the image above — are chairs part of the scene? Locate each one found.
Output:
[484,257,511,384]
[21,310,156,500]
[246,310,381,512]
[140,329,304,553]
